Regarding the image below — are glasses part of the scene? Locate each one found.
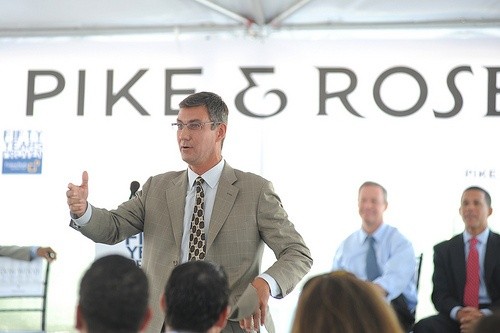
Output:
[171,122,222,134]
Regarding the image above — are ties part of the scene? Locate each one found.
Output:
[365,235,382,281]
[464,238,481,310]
[188,177,207,263]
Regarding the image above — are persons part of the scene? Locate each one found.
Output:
[289,271,403,333]
[75,254,152,333]
[0,245,57,264]
[412,187,500,333]
[159,261,232,333]
[330,181,418,333]
[66,92,313,333]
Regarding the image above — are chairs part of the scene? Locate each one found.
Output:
[0,245,56,333]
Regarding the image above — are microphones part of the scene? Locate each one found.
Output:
[129,181,141,199]
